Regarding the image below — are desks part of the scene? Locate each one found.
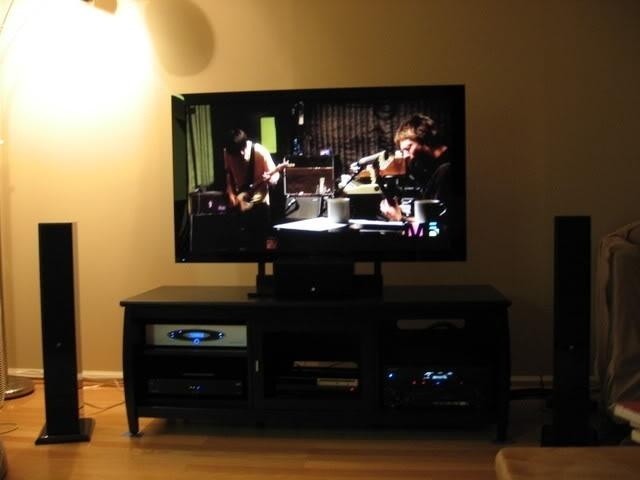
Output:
[492,443,640,480]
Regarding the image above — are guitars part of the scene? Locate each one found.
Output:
[226,160,295,217]
[373,158,414,222]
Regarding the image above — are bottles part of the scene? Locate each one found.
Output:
[293,139,300,156]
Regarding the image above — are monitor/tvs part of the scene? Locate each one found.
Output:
[171,84,467,302]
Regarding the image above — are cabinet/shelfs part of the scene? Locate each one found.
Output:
[118,284,512,448]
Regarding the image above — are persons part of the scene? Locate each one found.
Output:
[223,128,281,218]
[379,112,460,236]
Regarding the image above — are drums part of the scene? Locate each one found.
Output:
[191,191,224,215]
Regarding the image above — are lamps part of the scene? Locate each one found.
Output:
[0,0,118,401]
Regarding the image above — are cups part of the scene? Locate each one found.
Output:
[327,197,350,223]
[415,200,440,223]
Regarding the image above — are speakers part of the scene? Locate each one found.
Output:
[540,216,632,444]
[34,222,96,445]
